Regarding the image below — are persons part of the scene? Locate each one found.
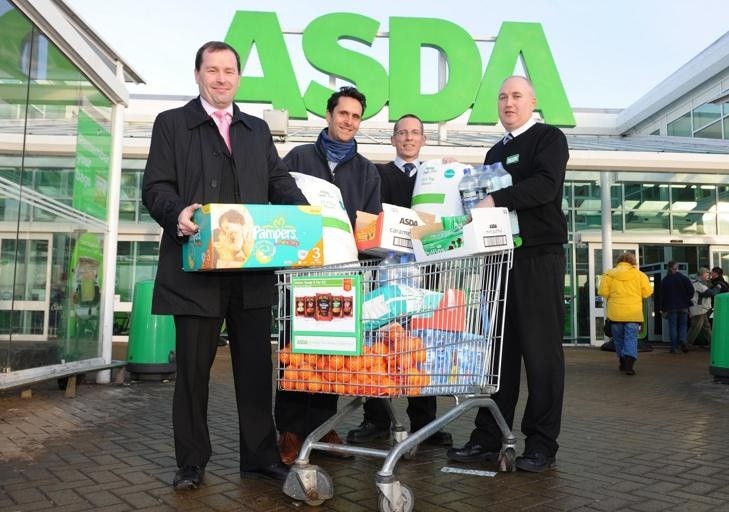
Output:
[447,75,572,475]
[688,267,712,348]
[218,210,255,258]
[274,86,385,467]
[346,114,458,457]
[213,231,245,268]
[597,250,654,377]
[140,38,312,491]
[703,266,729,298]
[657,260,694,354]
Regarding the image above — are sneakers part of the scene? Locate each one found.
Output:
[670,348,679,353]
[680,344,689,353]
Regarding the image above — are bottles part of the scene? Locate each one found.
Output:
[407,329,492,395]
[458,162,519,240]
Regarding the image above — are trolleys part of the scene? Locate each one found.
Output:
[270,233,520,511]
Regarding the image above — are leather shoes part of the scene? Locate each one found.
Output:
[447,442,499,462]
[410,425,455,446]
[516,453,555,474]
[277,432,300,464]
[319,429,350,458]
[172,468,205,489]
[240,461,290,480]
[346,422,391,443]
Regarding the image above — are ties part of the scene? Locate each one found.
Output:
[213,111,233,155]
[403,164,415,176]
[502,135,512,144]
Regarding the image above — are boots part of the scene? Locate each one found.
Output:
[619,356,635,375]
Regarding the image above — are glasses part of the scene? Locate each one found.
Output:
[397,130,421,138]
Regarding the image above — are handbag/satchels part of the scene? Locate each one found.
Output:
[604,320,612,338]
